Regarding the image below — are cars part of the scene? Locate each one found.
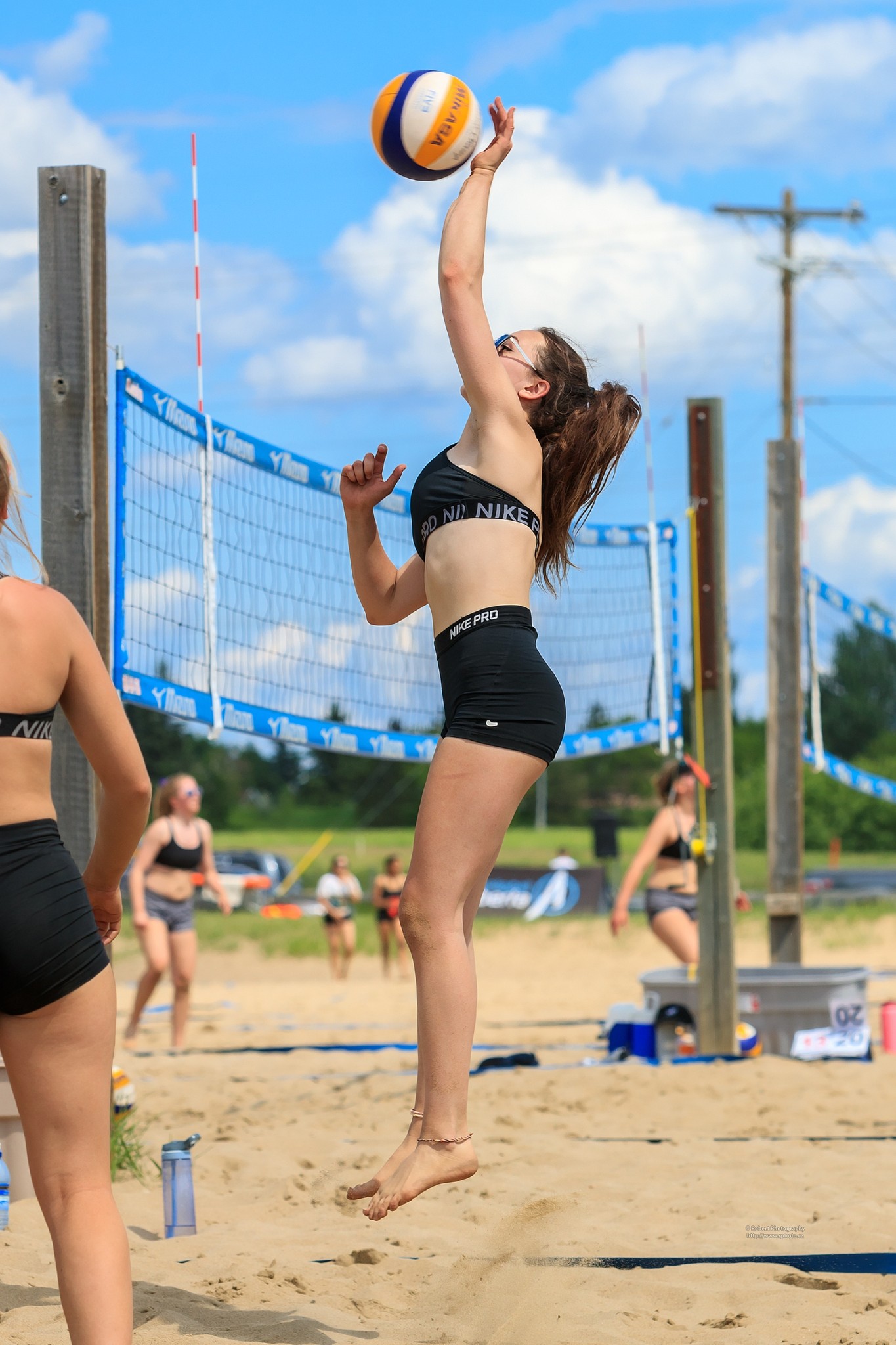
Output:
[198,848,302,916]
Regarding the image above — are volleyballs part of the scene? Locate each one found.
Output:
[370,70,481,182]
[737,1021,762,1059]
[111,1065,135,1124]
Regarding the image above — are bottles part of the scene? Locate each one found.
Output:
[161,1133,201,1238]
[0,1151,10,1231]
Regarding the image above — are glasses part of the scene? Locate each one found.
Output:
[494,334,547,407]
[186,785,205,798]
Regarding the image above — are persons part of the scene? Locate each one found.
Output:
[317,855,362,981]
[608,758,751,964]
[338,94,644,1219]
[0,436,153,1345]
[546,801,620,900]
[122,771,231,1052]
[371,854,408,982]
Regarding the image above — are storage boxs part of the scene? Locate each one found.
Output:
[639,966,867,1059]
